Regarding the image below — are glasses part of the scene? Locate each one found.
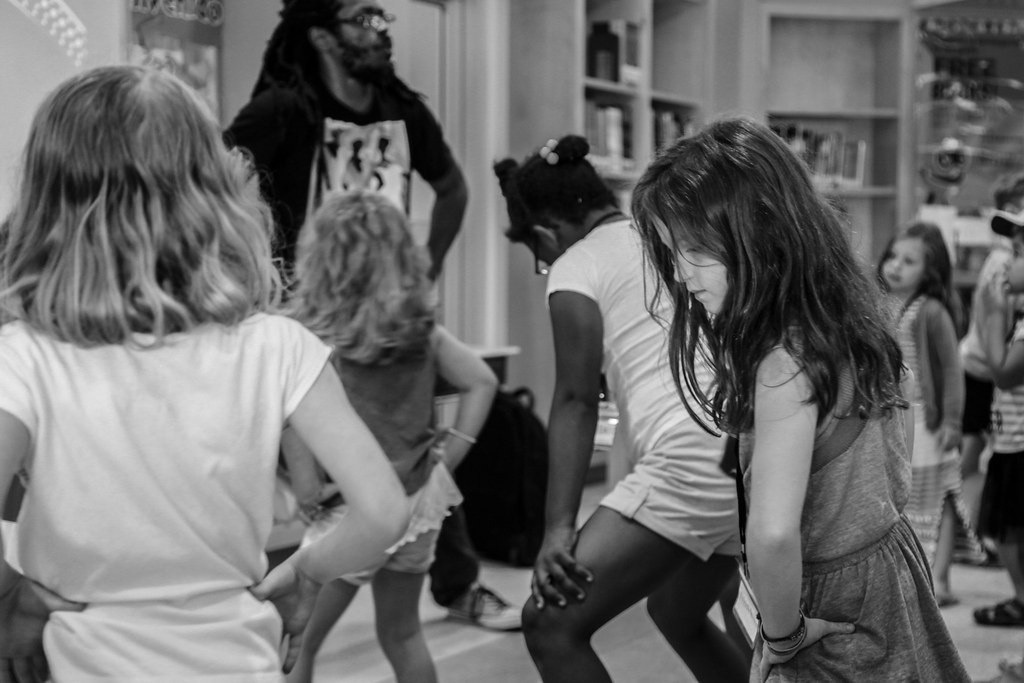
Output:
[534,225,558,275]
[329,13,392,28]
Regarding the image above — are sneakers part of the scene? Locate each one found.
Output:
[447,586,524,630]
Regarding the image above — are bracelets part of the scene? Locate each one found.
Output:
[760,609,808,655]
[447,427,476,443]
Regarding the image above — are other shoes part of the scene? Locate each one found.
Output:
[934,595,957,607]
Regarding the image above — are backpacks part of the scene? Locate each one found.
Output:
[454,379,549,568]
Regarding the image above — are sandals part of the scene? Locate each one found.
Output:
[974,598,1024,626]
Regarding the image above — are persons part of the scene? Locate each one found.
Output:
[218,0,522,629]
[880,176,1024,629]
[0,64,406,683]
[639,116,972,682]
[493,134,746,683]
[273,189,498,683]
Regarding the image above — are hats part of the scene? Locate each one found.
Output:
[990,210,1024,237]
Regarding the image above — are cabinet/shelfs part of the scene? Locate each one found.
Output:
[507,0,918,484]
